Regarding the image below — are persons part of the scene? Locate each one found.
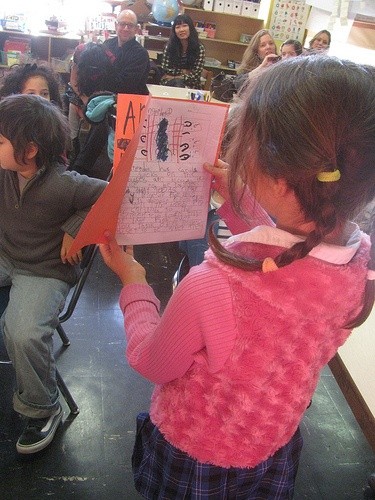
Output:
[98,56,375,500]
[0,95,110,454]
[158,14,205,90]
[66,10,150,182]
[0,63,61,103]
[235,30,331,92]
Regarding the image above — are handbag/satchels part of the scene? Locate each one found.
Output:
[209,71,237,103]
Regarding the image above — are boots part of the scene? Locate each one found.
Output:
[70,136,80,164]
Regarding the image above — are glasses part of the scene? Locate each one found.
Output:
[114,20,138,30]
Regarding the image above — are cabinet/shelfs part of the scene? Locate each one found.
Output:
[179,8,264,92]
[0,31,82,78]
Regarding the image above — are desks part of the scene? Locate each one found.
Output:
[146,84,242,112]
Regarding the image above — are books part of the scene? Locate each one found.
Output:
[63,93,230,260]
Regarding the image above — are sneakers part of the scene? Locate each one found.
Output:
[16,405,63,454]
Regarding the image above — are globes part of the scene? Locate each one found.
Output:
[151,0,179,26]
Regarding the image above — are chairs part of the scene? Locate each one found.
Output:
[0,166,113,416]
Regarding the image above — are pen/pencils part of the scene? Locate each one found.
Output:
[191,91,214,101]
